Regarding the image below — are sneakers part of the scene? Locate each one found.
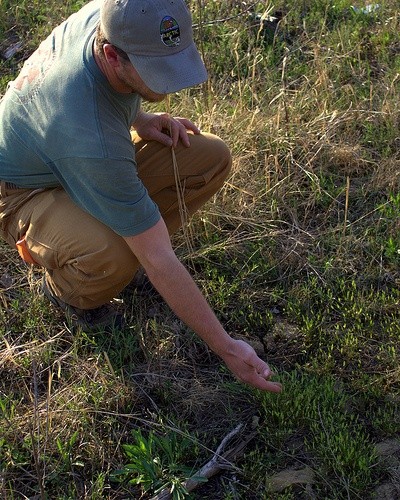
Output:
[41,271,113,335]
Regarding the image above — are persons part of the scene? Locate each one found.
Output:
[0,0,285,394]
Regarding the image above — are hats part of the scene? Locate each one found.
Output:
[99,0,208,95]
